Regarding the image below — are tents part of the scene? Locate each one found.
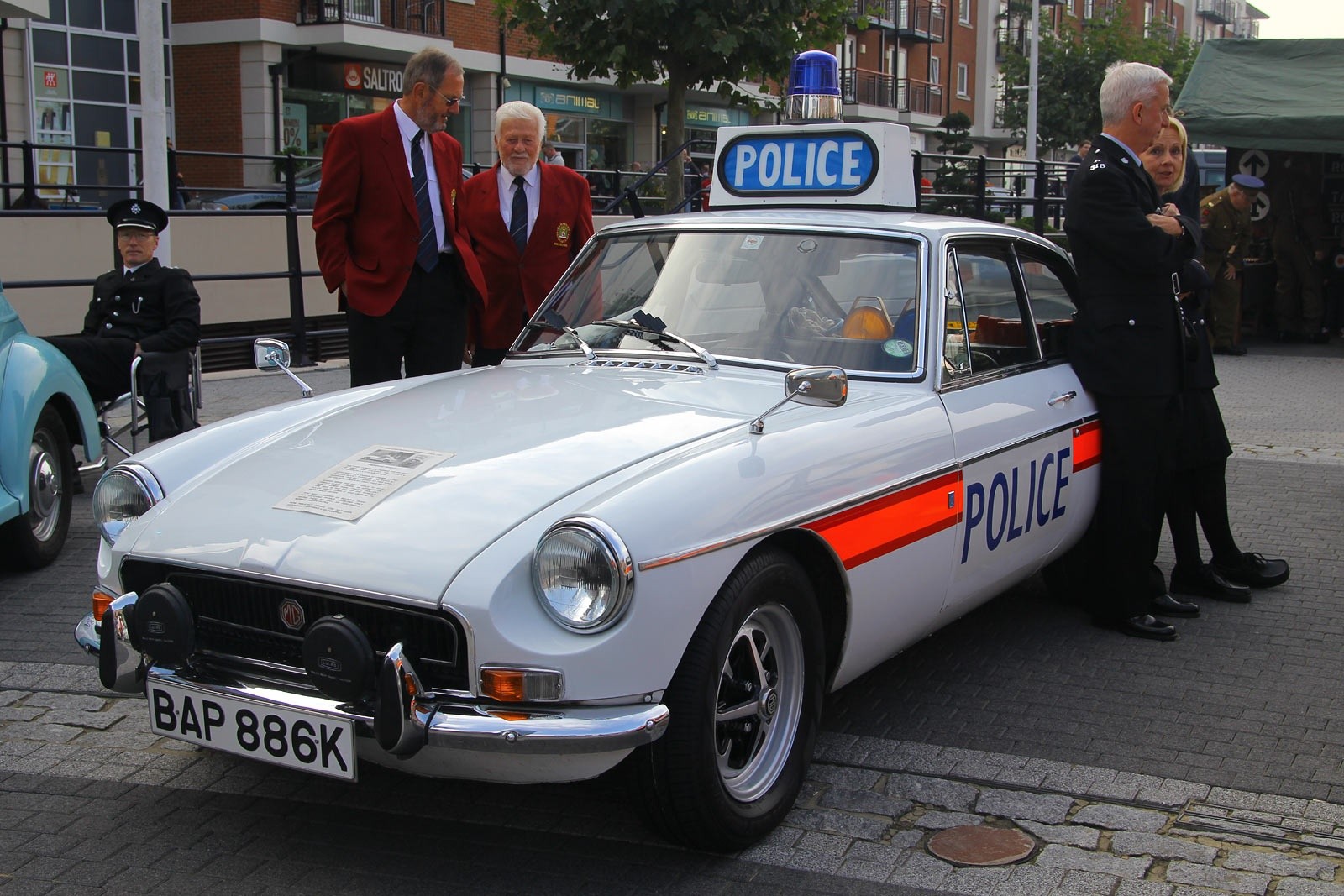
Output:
[1173,39,1344,154]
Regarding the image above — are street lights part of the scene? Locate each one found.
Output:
[1023,0,1065,219]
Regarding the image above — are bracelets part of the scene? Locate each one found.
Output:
[1178,221,1185,238]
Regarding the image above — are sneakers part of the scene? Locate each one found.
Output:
[1170,564,1252,603]
[1210,551,1290,587]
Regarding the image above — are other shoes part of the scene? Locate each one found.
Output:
[1214,346,1247,355]
[73,476,86,494]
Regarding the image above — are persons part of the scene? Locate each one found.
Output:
[1068,61,1200,640]
[1138,116,1290,602]
[585,164,606,196]
[684,149,700,212]
[620,162,641,197]
[311,49,488,389]
[541,143,565,166]
[1264,152,1331,345]
[461,99,603,366]
[35,200,201,400]
[701,164,713,211]
[1066,140,1092,192]
[920,173,931,199]
[167,137,186,209]
[1199,173,1264,356]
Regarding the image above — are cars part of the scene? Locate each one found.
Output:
[77,46,1109,851]
[983,184,1016,216]
[0,285,110,571]
[185,158,473,211]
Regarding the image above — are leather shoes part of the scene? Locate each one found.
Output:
[1147,594,1200,618]
[1092,614,1176,641]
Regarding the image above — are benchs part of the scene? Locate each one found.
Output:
[806,337,914,373]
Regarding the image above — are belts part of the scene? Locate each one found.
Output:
[1078,271,1181,299]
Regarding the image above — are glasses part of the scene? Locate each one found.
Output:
[425,83,465,106]
[119,232,154,241]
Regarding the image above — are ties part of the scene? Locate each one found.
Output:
[411,128,440,274]
[124,270,132,282]
[510,177,527,252]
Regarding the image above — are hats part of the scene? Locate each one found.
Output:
[1232,173,1265,202]
[107,199,168,236]
